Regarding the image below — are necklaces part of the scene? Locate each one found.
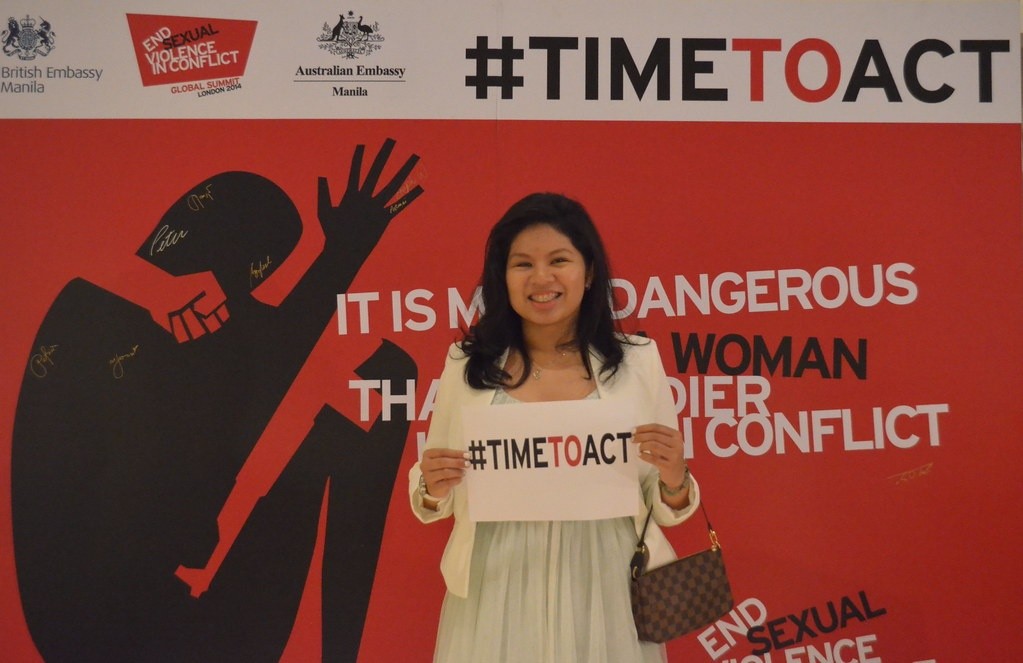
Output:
[532,353,566,380]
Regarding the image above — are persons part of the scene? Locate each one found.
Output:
[408,193,701,663]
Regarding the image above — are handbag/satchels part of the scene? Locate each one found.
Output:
[630,498,734,644]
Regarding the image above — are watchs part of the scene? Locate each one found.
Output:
[418,475,439,502]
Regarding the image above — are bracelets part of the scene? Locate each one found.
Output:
[658,464,690,495]
